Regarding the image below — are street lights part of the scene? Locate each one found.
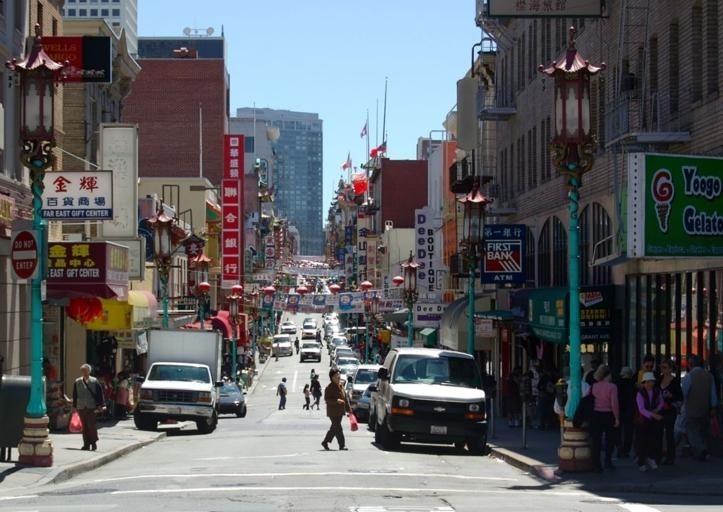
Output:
[227,291,242,378]
[146,197,176,328]
[459,174,493,353]
[401,248,421,346]
[250,291,260,375]
[192,252,210,328]
[537,22,610,472]
[7,19,70,467]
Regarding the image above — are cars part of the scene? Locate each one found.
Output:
[216,382,246,417]
[268,274,489,455]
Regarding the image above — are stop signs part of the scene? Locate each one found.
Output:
[9,229,39,282]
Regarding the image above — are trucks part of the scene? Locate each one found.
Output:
[132,329,224,433]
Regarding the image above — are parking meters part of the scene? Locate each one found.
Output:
[554,378,568,448]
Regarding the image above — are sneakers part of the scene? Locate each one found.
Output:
[591,459,617,474]
[646,456,659,471]
[79,441,98,451]
[638,464,650,472]
[320,440,348,452]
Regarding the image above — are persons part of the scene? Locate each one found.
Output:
[481,348,722,475]
[310,369,315,380]
[276,377,287,410]
[308,375,321,409]
[42,357,58,381]
[302,384,311,410]
[114,371,130,418]
[319,368,352,450]
[72,363,106,452]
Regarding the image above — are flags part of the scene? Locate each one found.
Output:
[359,123,366,139]
[353,179,368,197]
[340,159,352,171]
[375,142,386,153]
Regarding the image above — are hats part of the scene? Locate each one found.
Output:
[555,378,568,386]
[640,371,657,384]
[619,366,634,377]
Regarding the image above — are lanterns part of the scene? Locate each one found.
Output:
[392,275,404,284]
[294,287,308,294]
[328,283,340,295]
[198,282,211,294]
[262,285,275,294]
[67,296,90,320]
[359,281,373,292]
[231,285,243,294]
[75,297,102,325]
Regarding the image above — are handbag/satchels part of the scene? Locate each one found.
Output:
[96,399,106,415]
[582,384,596,422]
